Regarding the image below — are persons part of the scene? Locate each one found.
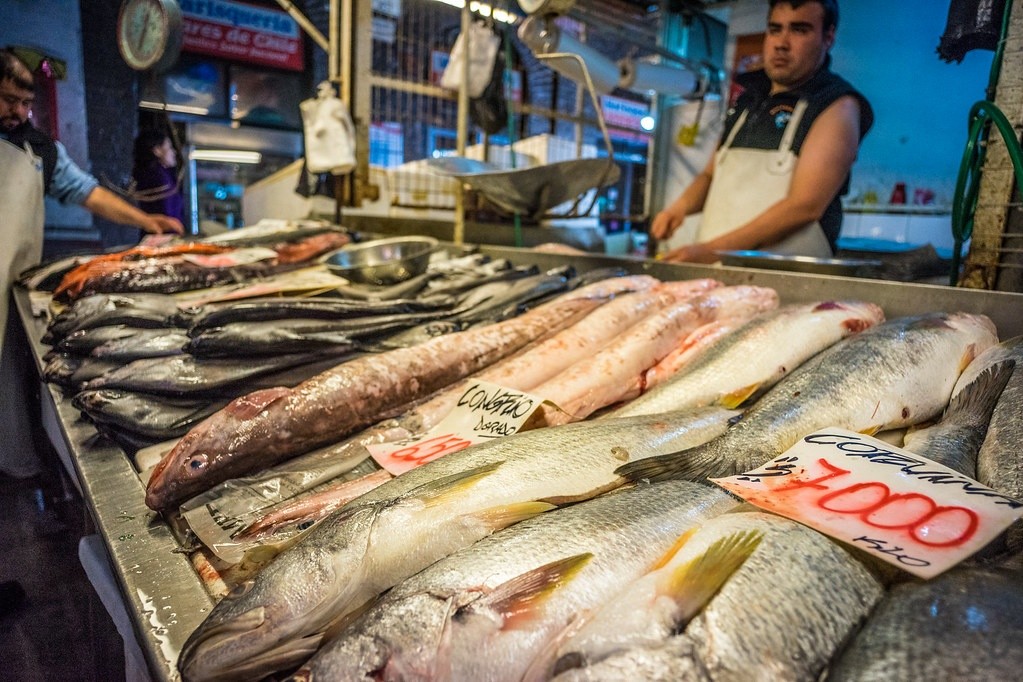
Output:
[128,129,187,244]
[1,51,184,616]
[647,0,875,265]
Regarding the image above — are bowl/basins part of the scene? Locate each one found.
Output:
[317,235,444,289]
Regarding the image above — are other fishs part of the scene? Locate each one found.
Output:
[16,217,1023,681]
